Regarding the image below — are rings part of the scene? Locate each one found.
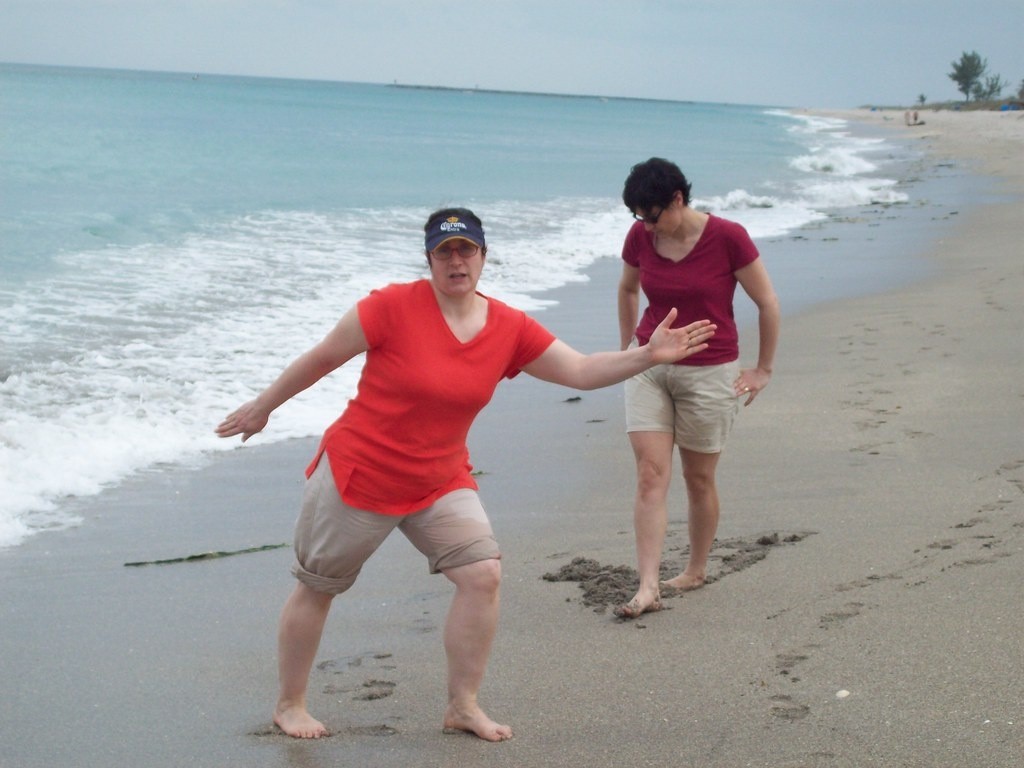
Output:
[689,340,691,347]
[744,387,749,392]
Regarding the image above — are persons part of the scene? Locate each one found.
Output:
[214,208,717,742]
[609,156,781,615]
[904,110,917,124]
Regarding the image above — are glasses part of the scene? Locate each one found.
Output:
[633,193,675,224]
[430,244,480,259]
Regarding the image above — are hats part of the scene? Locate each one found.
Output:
[425,214,484,253]
